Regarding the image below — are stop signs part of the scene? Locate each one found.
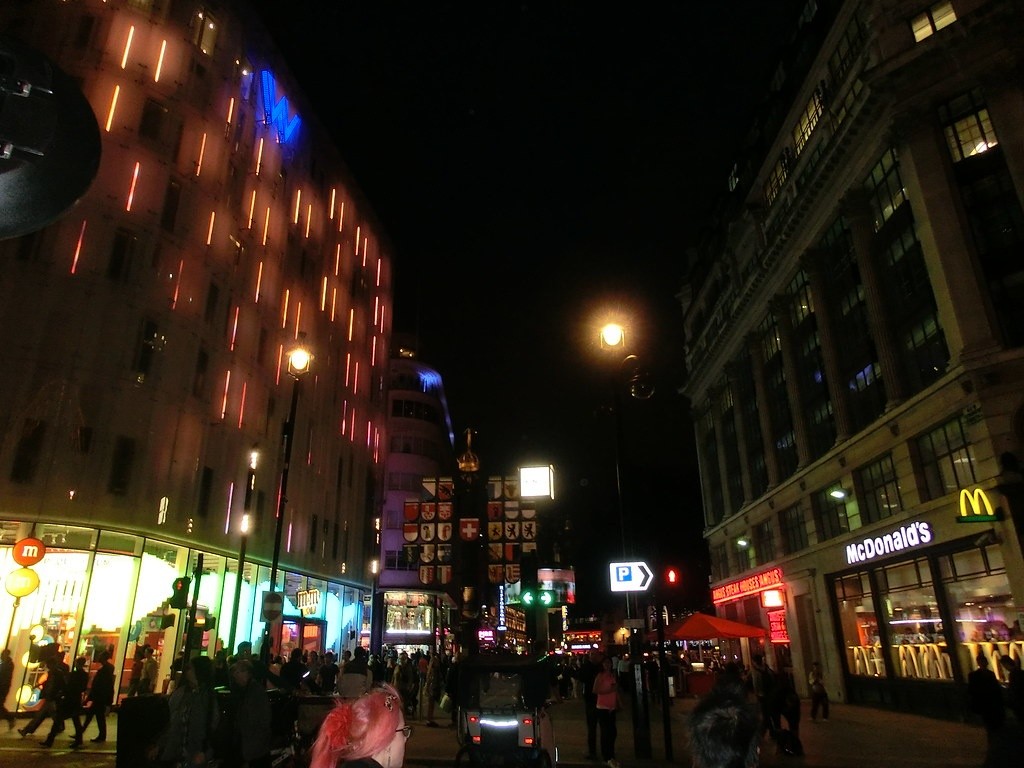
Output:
[260,592,283,623]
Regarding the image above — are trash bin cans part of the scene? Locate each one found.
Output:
[115,692,171,768]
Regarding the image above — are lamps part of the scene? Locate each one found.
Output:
[829,487,853,498]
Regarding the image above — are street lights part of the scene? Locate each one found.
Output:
[591,308,656,763]
[262,341,315,689]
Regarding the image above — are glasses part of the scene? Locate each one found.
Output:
[395,724,413,738]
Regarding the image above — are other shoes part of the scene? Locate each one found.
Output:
[39,741,51,748]
[18,729,27,737]
[69,735,75,739]
[90,739,105,743]
[8,717,16,729]
[427,722,438,727]
[69,743,80,748]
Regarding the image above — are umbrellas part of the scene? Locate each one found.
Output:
[647,612,768,662]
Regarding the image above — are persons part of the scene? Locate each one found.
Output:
[773,669,805,756]
[0,645,691,768]
[385,605,429,632]
[746,653,785,755]
[684,689,762,768]
[809,661,831,724]
[712,661,747,704]
[873,620,1024,768]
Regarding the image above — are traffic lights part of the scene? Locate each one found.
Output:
[652,561,681,596]
[169,576,190,609]
[518,589,555,608]
